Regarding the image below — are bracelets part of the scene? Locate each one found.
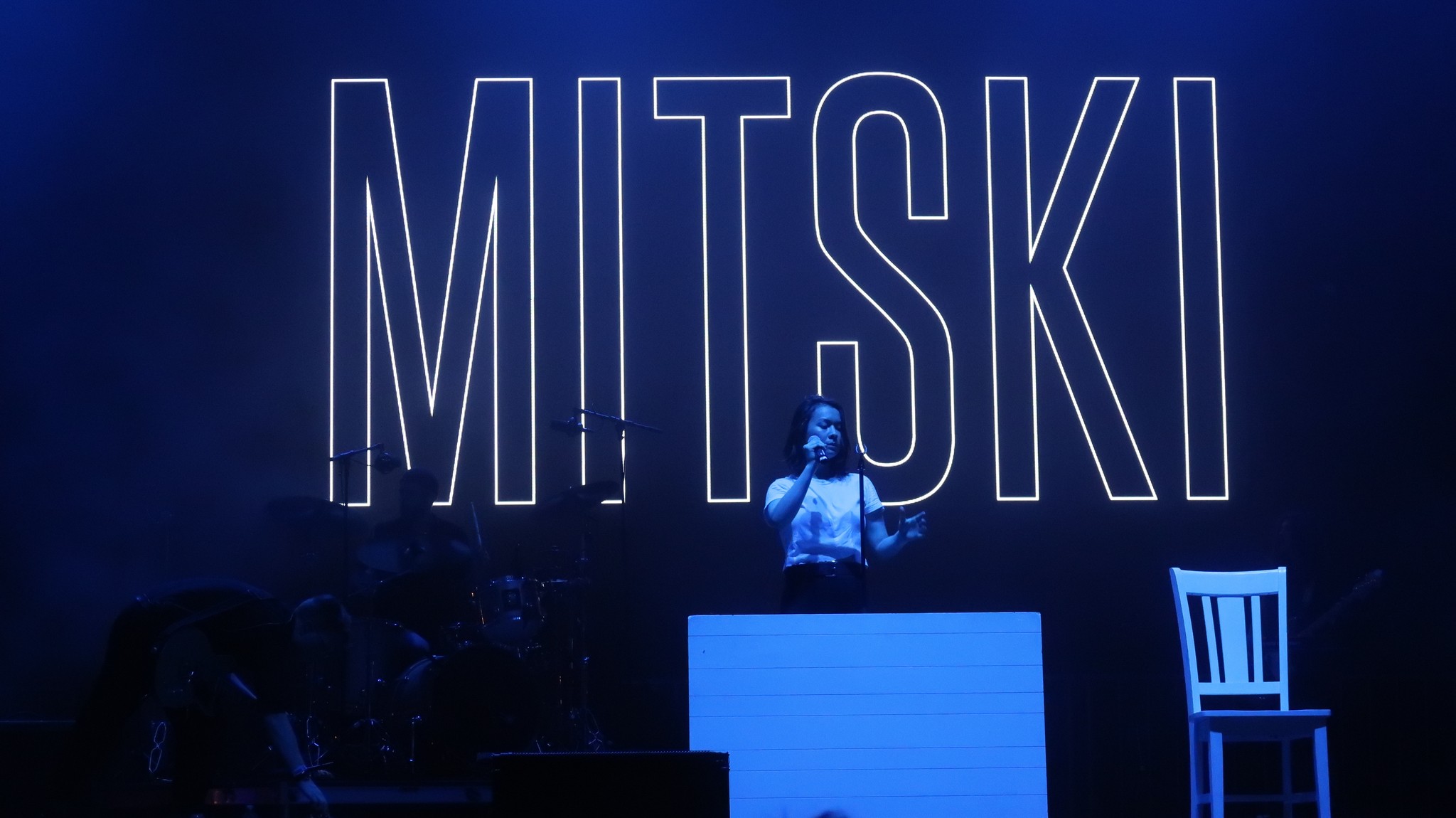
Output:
[291,768,310,785]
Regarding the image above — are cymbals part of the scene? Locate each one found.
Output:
[524,480,620,518]
[541,578,567,583]
[265,495,342,521]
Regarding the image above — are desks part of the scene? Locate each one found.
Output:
[685,604,1050,818]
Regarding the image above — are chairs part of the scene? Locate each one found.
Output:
[1168,566,1333,818]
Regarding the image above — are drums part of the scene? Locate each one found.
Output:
[339,618,401,684]
[470,575,544,649]
[392,654,448,748]
[429,622,485,654]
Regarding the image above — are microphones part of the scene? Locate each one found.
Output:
[808,435,827,462]
[553,416,598,436]
[374,452,401,474]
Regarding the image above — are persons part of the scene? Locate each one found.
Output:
[366,472,474,613]
[166,593,341,814]
[761,393,929,615]
